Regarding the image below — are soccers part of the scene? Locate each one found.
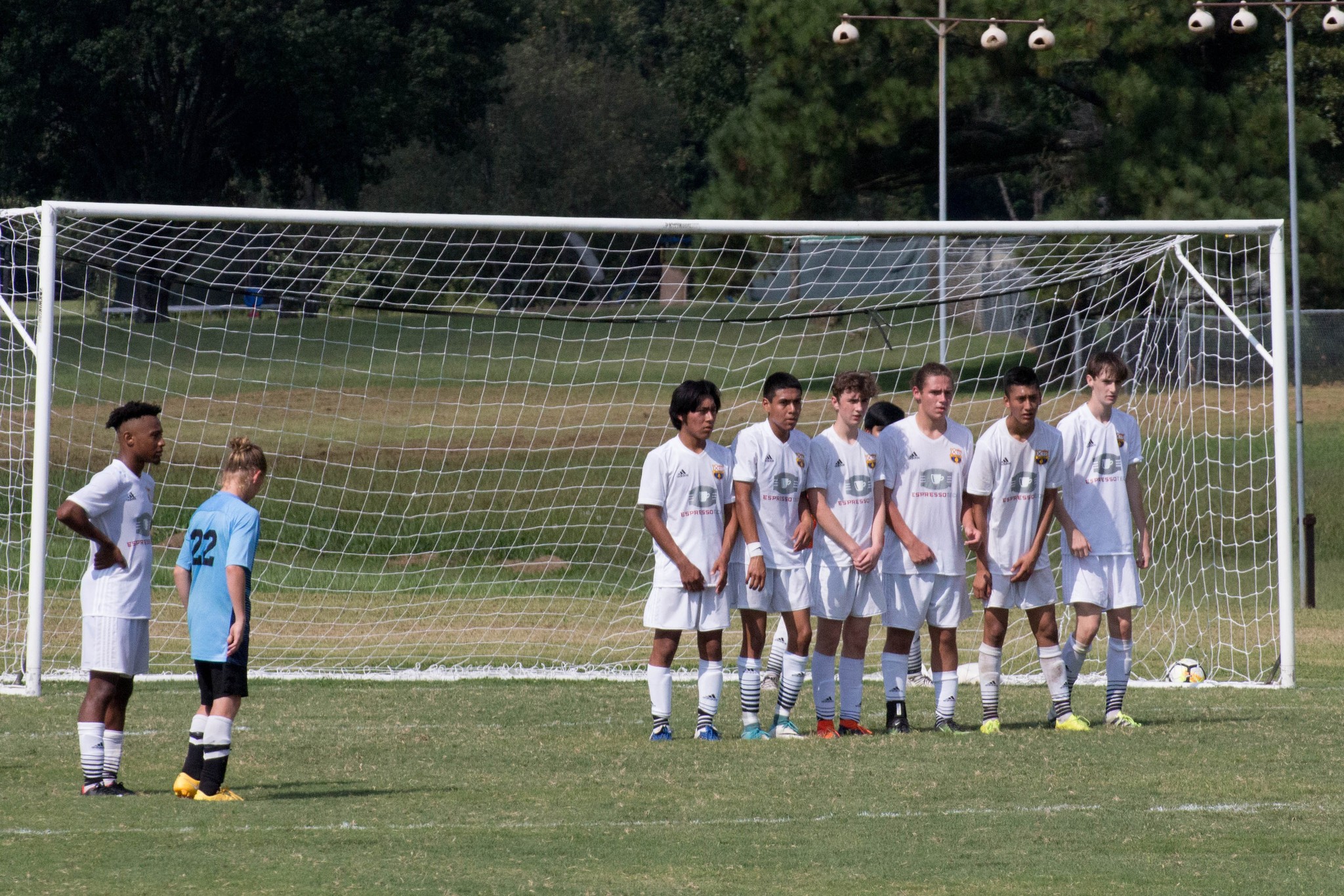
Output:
[1167,658,1205,687]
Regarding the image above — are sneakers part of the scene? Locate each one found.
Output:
[838,720,875,737]
[1048,707,1057,728]
[933,719,975,735]
[106,780,135,795]
[741,728,771,741]
[1103,710,1142,729]
[1055,714,1092,732]
[694,724,722,741]
[768,720,805,740]
[886,721,916,736]
[649,725,672,742]
[817,720,840,738]
[906,676,934,689]
[194,787,244,801]
[80,782,116,797]
[173,772,200,798]
[980,719,1000,735]
[760,672,780,692]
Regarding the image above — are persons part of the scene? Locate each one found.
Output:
[637,379,740,740]
[1047,351,1152,729]
[879,361,984,735]
[172,437,267,801]
[56,400,165,795]
[965,366,1091,733]
[729,372,935,741]
